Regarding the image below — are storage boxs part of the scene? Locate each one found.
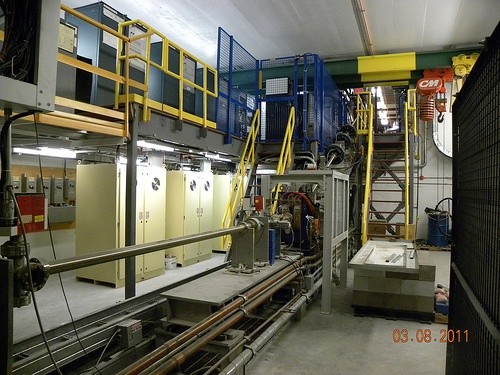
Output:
[368,220,387,238]
[396,222,416,240]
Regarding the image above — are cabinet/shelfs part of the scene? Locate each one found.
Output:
[213,175,249,253]
[164,170,214,268]
[75,163,166,289]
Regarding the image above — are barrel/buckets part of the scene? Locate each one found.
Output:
[426,214,449,247]
